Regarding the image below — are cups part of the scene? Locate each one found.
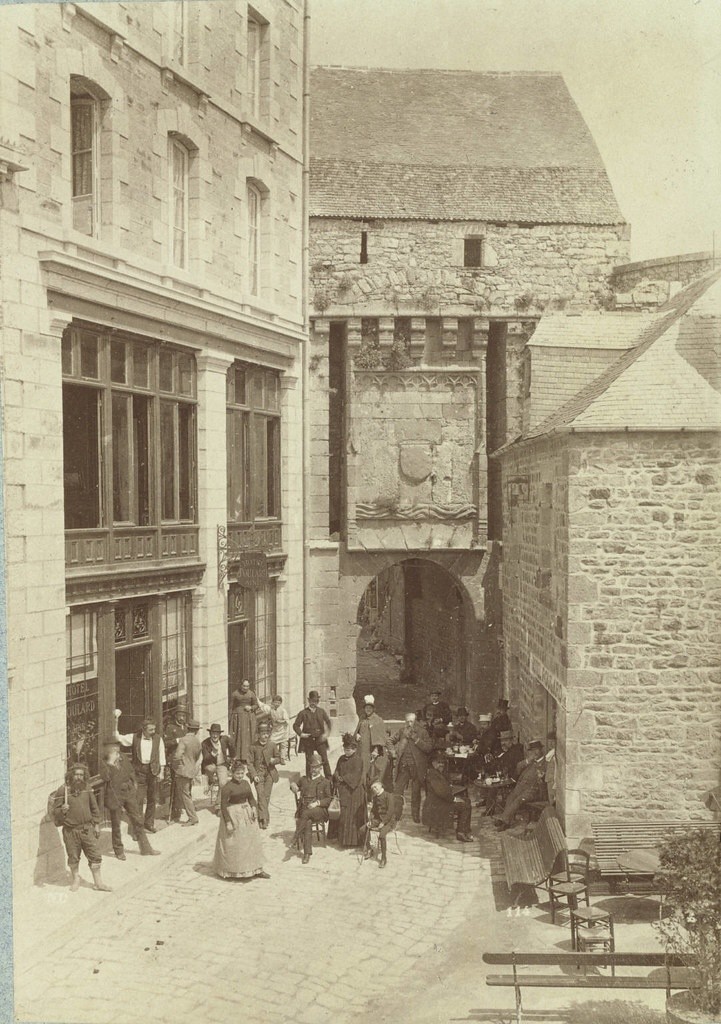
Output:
[485,777,493,786]
[445,747,452,755]
[453,745,459,751]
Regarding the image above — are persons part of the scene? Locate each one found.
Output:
[246,723,281,830]
[289,731,371,864]
[53,762,114,892]
[201,723,237,817]
[292,691,335,779]
[164,705,203,826]
[99,736,161,861]
[214,764,271,881]
[229,678,290,765]
[354,690,524,868]
[494,740,549,833]
[112,709,166,841]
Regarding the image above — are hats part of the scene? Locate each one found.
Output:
[307,691,321,699]
[369,776,381,788]
[171,704,270,734]
[431,687,544,752]
[308,755,324,766]
[103,735,121,745]
[362,695,375,708]
[342,732,357,749]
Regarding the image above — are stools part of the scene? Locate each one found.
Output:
[577,928,616,977]
[570,906,615,952]
[548,881,589,927]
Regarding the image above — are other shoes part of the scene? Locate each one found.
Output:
[290,838,297,849]
[457,796,509,842]
[255,871,271,878]
[302,853,310,863]
[93,883,112,892]
[394,814,400,821]
[69,876,80,892]
[379,857,386,868]
[114,805,269,860]
[364,848,373,860]
[413,814,419,823]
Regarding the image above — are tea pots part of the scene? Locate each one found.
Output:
[459,744,467,754]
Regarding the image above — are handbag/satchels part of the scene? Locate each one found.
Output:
[328,786,341,820]
[48,783,67,827]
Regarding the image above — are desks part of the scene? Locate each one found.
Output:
[474,777,514,816]
[439,748,470,760]
[272,734,298,760]
[449,783,468,796]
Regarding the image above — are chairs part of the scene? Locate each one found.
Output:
[549,849,590,910]
[290,786,326,849]
[366,795,407,859]
[424,758,467,840]
[210,774,220,804]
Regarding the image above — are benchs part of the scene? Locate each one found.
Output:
[500,805,568,909]
[591,819,721,893]
[482,952,701,1024]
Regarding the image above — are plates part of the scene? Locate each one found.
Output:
[493,778,500,783]
